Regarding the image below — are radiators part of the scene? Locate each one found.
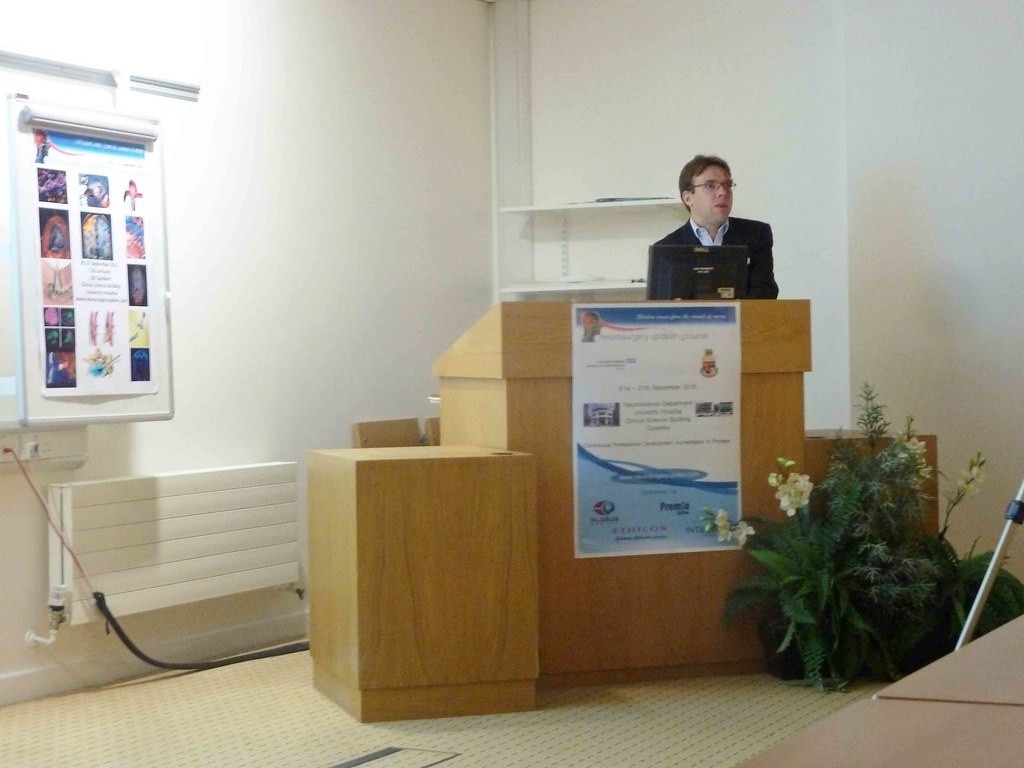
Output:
[24,462,305,648]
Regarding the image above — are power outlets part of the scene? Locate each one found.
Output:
[0,433,19,464]
[36,432,60,459]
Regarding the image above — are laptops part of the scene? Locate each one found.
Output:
[647,246,749,300]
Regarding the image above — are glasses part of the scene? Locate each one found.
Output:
[686,180,737,192]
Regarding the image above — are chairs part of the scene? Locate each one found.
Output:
[354,418,423,448]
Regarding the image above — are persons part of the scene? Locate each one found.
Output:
[645,154,779,300]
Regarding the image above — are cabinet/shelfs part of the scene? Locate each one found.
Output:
[499,198,683,291]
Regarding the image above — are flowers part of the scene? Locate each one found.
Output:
[700,381,1024,695]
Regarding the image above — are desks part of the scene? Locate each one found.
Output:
[723,612,1024,767]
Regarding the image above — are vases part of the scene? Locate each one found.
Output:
[756,619,830,681]
[879,614,951,677]
